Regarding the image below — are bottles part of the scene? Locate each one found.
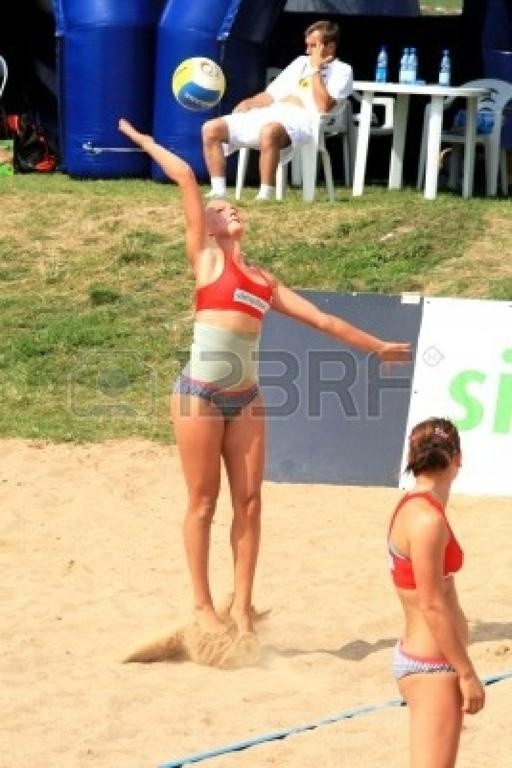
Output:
[440,50,451,86]
[400,47,417,83]
[375,47,389,80]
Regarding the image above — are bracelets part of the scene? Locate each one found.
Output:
[309,69,321,75]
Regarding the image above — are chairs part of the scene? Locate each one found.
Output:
[416,77,512,198]
[326,90,395,190]
[221,100,349,202]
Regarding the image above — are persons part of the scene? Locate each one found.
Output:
[200,19,355,200]
[384,414,485,768]
[118,116,414,635]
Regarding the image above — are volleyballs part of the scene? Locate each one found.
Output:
[172,57,226,111]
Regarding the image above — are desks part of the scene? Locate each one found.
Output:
[350,80,489,202]
